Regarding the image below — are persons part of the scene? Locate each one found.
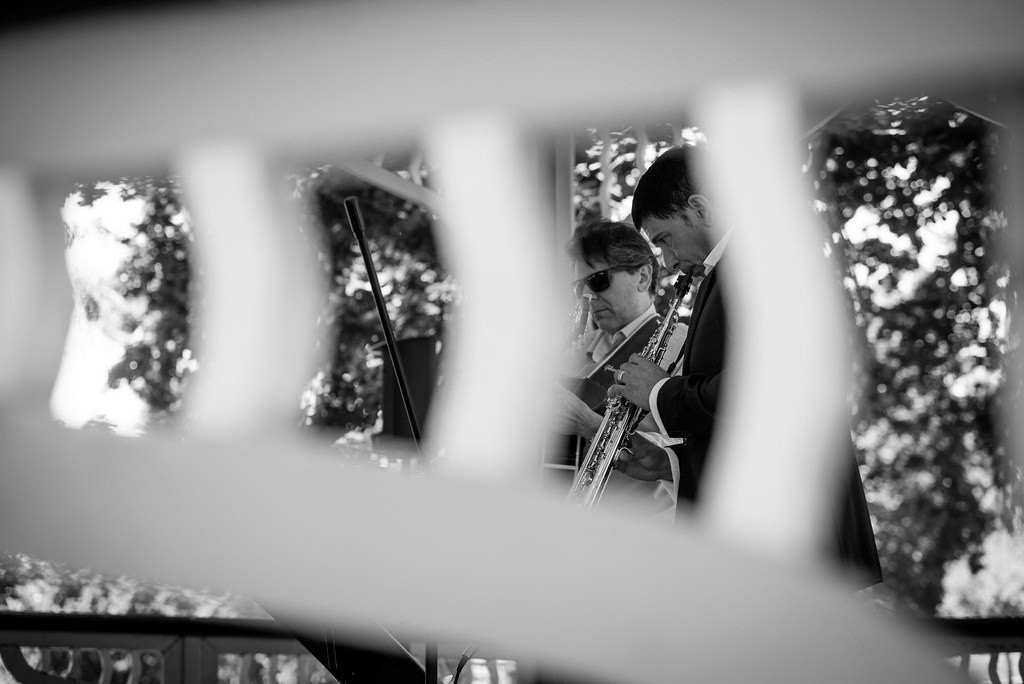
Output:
[609,144,883,596]
[548,217,665,475]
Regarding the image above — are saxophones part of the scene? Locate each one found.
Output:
[566,270,695,505]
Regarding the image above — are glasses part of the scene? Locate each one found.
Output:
[572,265,619,299]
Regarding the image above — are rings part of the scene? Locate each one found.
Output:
[618,371,625,383]
